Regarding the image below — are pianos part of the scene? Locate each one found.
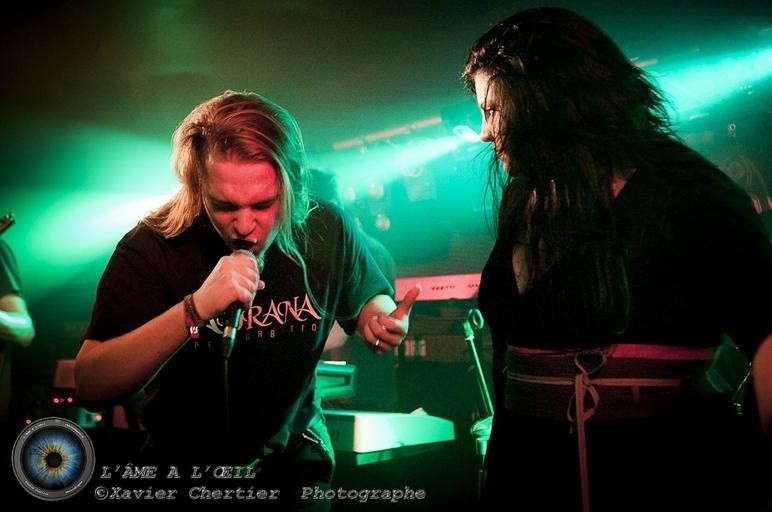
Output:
[321,405,457,478]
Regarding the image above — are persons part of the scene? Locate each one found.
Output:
[459,7,772,512]
[73,89,422,512]
[0,237,36,411]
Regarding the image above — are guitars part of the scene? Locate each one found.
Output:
[457,318,495,477]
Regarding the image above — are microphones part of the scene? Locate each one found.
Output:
[221,247,255,359]
